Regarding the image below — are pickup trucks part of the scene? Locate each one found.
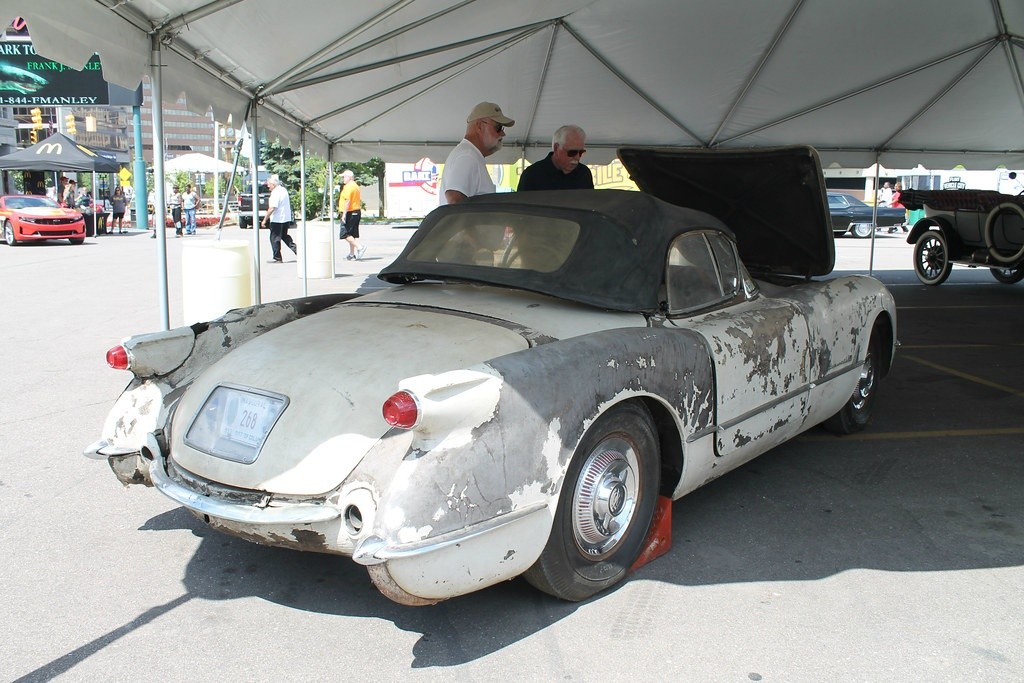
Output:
[238,180,295,229]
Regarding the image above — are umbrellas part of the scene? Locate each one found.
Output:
[146,153,249,199]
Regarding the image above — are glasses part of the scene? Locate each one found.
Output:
[564,148,586,157]
[482,121,504,133]
[116,190,119,191]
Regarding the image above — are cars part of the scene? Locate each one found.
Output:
[826,192,906,238]
[86,143,901,610]
[0,195,86,247]
[897,186,1024,285]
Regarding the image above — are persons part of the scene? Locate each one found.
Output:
[438,102,515,206]
[169,184,201,238]
[517,125,594,192]
[103,185,130,234]
[338,170,367,262]
[877,182,908,234]
[45,176,93,209]
[261,174,297,264]
[148,187,156,239]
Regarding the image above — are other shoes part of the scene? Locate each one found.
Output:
[150,234,156,238]
[184,233,191,235]
[119,231,122,234]
[266,260,283,263]
[356,245,367,260]
[343,254,356,261]
[192,231,196,235]
[107,231,113,234]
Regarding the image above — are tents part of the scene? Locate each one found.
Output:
[0,132,120,239]
[0,0,1024,332]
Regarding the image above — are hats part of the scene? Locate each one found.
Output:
[339,170,354,176]
[69,180,77,183]
[60,177,69,180]
[467,102,515,127]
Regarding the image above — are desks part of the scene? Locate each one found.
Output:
[82,213,110,237]
